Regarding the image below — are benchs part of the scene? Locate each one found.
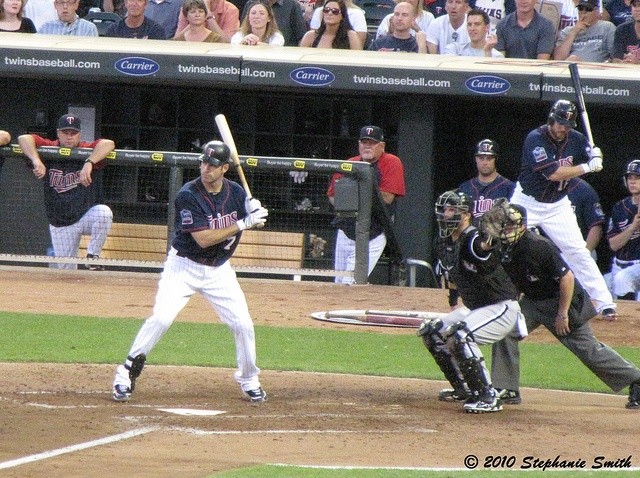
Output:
[78,222,304,282]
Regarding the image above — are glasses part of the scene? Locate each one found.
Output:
[578,7,593,12]
[56,1,75,6]
[323,7,339,15]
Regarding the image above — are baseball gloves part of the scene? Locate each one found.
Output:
[477,199,509,246]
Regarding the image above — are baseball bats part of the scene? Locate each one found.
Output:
[567,61,600,171]
[215,111,265,229]
[326,313,426,326]
[365,309,447,318]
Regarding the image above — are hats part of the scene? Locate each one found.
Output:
[359,126,384,143]
[57,114,82,131]
[576,0,600,9]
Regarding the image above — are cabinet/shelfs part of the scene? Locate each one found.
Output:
[98,82,397,218]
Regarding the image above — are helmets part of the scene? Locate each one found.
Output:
[549,99,578,128]
[198,140,230,168]
[434,188,475,238]
[498,204,527,263]
[474,139,499,157]
[624,160,640,175]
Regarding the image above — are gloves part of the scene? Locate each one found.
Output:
[245,196,261,215]
[237,209,268,232]
[581,158,603,173]
[585,146,603,159]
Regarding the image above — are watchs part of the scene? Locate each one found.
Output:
[84,157,96,166]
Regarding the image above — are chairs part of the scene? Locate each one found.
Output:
[360,0,396,20]
[82,12,122,37]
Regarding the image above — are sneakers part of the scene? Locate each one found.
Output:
[625,379,640,408]
[87,254,103,270]
[438,383,472,403]
[113,385,131,402]
[464,388,503,413]
[246,388,267,402]
[603,309,617,318]
[497,388,521,404]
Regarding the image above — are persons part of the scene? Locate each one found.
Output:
[444,9,505,57]
[240,0,308,46]
[367,1,427,53]
[509,98,619,319]
[554,0,603,31]
[0,129,12,170]
[468,0,516,33]
[38,0,99,36]
[491,203,639,408]
[553,0,616,63]
[310,0,367,46]
[231,2,285,46]
[495,0,555,60]
[417,190,521,413]
[0,0,37,33]
[615,0,640,64]
[18,114,114,271]
[144,0,180,39]
[174,0,223,42]
[608,0,634,24]
[327,126,405,284]
[105,0,166,40]
[426,0,471,53]
[607,159,640,300]
[458,138,516,223]
[566,175,606,261]
[300,0,363,50]
[112,140,268,402]
[376,0,434,39]
[176,0,240,43]
